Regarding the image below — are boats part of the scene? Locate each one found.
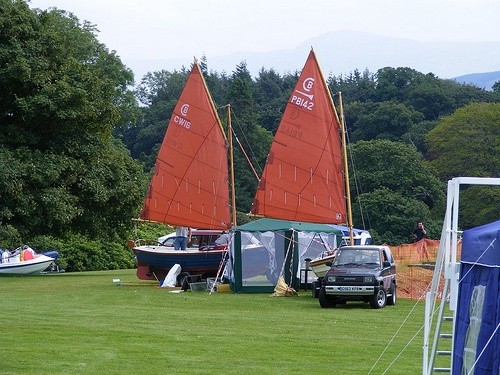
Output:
[0,244,60,276]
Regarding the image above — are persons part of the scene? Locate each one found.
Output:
[412,221,432,265]
[166,224,193,251]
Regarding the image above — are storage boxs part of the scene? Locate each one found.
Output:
[185,274,220,292]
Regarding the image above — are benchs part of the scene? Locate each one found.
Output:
[20,250,33,261]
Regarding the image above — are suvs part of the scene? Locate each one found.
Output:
[320,244,397,309]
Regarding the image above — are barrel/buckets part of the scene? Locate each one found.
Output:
[112,278,120,287]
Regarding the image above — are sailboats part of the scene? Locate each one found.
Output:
[132,60,271,281]
[243,46,371,249]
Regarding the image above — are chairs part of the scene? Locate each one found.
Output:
[371,252,380,262]
